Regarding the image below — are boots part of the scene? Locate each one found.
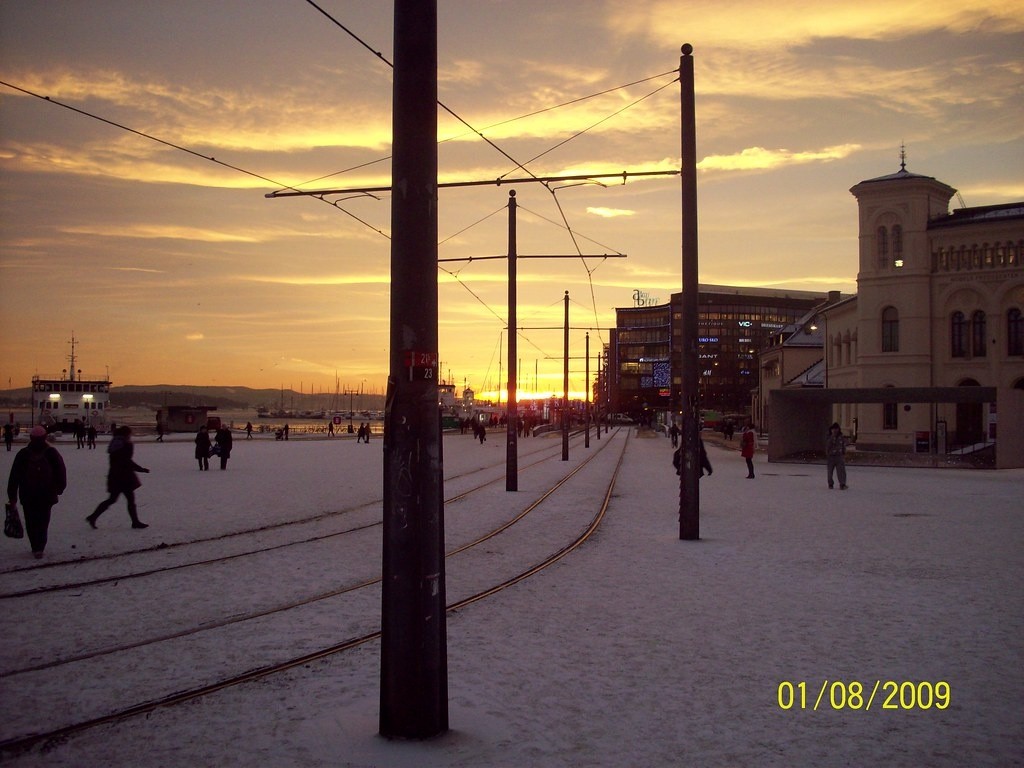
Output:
[86,503,108,529]
[128,505,149,529]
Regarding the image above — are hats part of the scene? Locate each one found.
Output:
[200,425,208,429]
[30,425,48,442]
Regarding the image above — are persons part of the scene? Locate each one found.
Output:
[274,426,283,441]
[3,425,67,560]
[86,425,150,530]
[720,420,736,441]
[823,421,849,490]
[2,421,14,451]
[328,421,336,438]
[154,421,164,443]
[357,422,372,444]
[215,423,233,470]
[283,423,289,441]
[488,414,507,428]
[86,425,98,450]
[740,423,758,479]
[194,425,211,471]
[459,415,487,444]
[669,423,681,449]
[243,422,253,439]
[518,414,542,438]
[72,423,86,450]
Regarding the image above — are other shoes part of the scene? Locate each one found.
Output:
[32,550,43,558]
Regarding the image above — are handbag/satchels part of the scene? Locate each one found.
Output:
[4,503,23,539]
[208,447,215,458]
